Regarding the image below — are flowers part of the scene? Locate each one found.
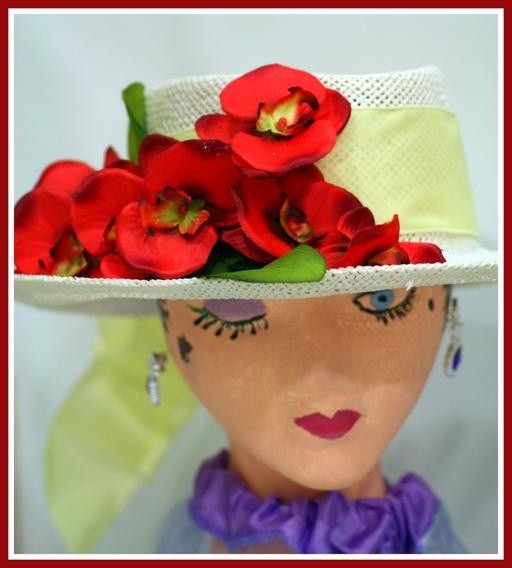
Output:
[16,59,452,278]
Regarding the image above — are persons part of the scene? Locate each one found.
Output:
[14,66,498,554]
